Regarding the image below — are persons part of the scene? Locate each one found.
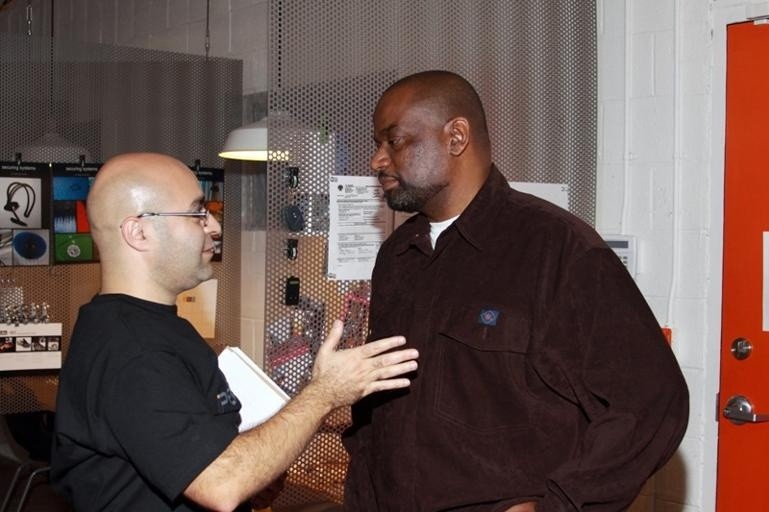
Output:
[343,70,688,511]
[50,151,419,512]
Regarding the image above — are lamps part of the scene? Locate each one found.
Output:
[216,0,313,164]
[7,1,96,172]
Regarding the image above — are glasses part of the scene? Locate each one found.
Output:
[137,209,211,227]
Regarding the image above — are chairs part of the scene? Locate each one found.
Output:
[0,407,58,512]
